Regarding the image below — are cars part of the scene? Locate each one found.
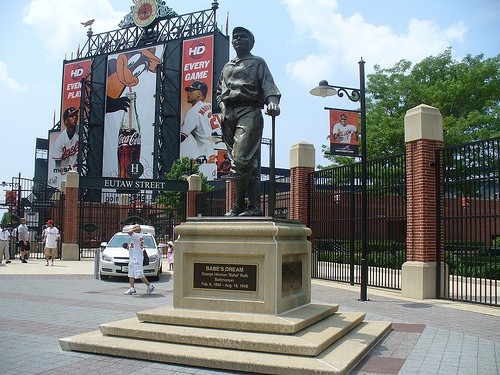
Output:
[99,224,163,281]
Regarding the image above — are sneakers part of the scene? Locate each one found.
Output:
[51,262,54,266]
[146,284,156,296]
[45,262,49,266]
[124,288,136,295]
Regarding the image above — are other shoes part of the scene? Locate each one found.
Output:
[0,262,1,263]
[6,261,11,264]
[22,259,28,263]
[20,256,22,260]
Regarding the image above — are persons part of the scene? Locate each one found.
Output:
[122,224,156,296]
[41,220,61,266]
[0,223,12,264]
[332,114,357,143]
[180,80,228,182]
[51,106,88,176]
[167,241,174,270]
[18,218,30,263]
[216,26,281,217]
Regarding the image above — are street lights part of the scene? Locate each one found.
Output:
[0,181,22,255]
[309,56,372,302]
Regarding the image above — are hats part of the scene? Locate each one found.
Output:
[48,220,54,224]
[167,241,174,247]
[185,80,207,95]
[63,107,79,121]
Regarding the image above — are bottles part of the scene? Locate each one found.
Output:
[117,92,141,178]
